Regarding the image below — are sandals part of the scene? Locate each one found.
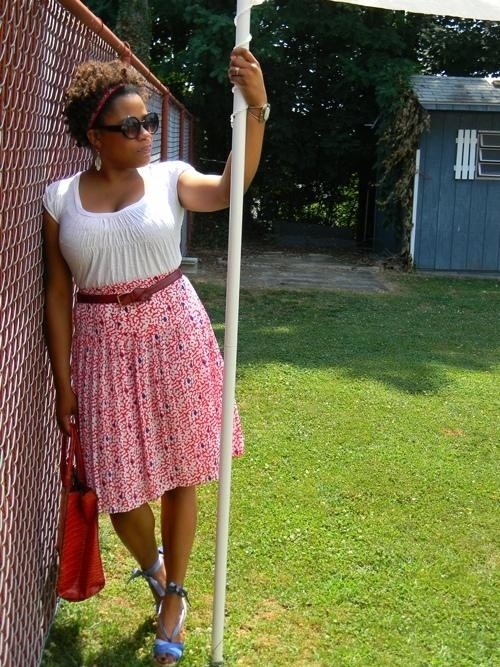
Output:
[140,549,167,608]
[153,586,187,665]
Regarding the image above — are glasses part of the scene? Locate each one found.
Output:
[104,113,160,139]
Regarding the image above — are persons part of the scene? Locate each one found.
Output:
[40,39,274,665]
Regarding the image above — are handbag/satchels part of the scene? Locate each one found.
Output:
[57,425,105,602]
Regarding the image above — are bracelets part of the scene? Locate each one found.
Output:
[247,100,272,123]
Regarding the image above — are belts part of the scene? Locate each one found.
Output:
[76,269,183,305]
